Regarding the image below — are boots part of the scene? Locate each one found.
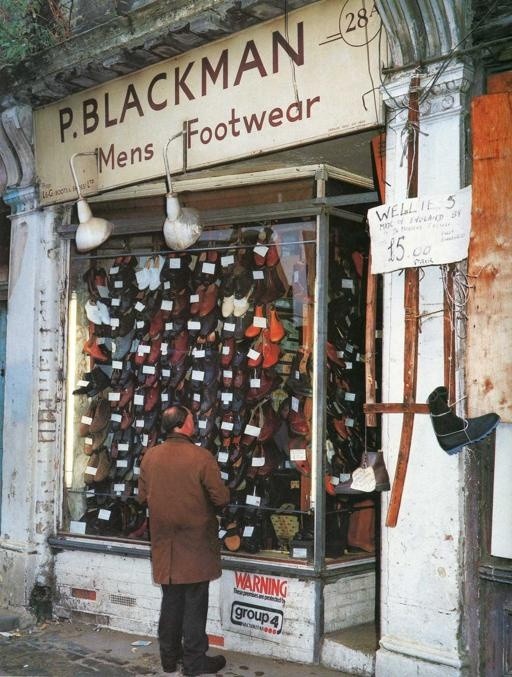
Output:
[335,451,390,495]
[425,386,501,456]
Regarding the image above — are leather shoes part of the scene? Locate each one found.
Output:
[163,645,226,676]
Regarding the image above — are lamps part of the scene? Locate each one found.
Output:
[161,131,204,251]
[69,148,114,252]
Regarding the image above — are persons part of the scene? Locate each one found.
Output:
[137,405,231,676]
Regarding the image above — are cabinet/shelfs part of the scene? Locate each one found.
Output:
[47,164,381,582]
